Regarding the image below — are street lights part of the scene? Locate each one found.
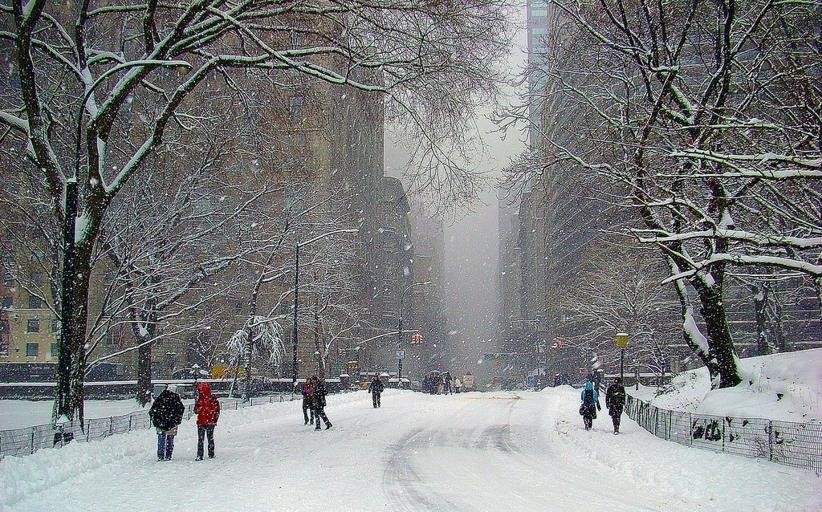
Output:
[291,227,361,392]
[398,282,432,381]
[52,59,194,448]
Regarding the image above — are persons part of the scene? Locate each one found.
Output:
[605,377,625,434]
[423,370,462,395]
[301,378,314,425]
[311,376,332,431]
[149,385,185,461]
[368,374,383,408]
[581,383,598,430]
[194,382,220,461]
[592,369,600,396]
